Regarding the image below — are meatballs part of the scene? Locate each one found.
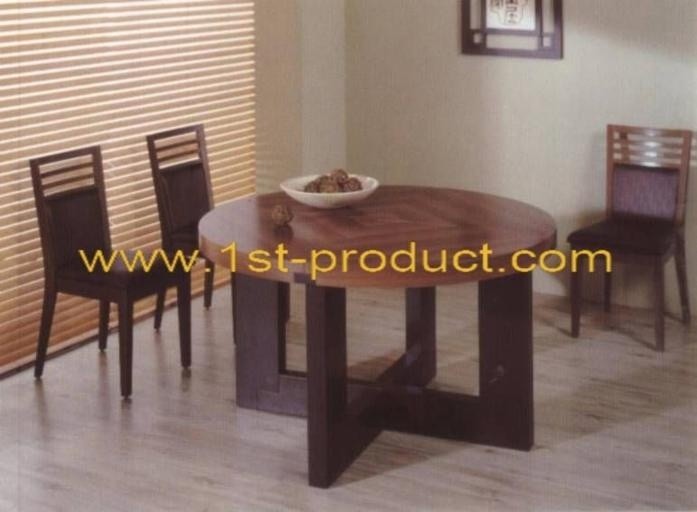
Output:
[272,204,293,226]
[304,169,361,192]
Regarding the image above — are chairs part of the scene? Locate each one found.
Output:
[566,124,694,352]
[28,124,237,398]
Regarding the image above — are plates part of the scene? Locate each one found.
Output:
[282,173,379,208]
